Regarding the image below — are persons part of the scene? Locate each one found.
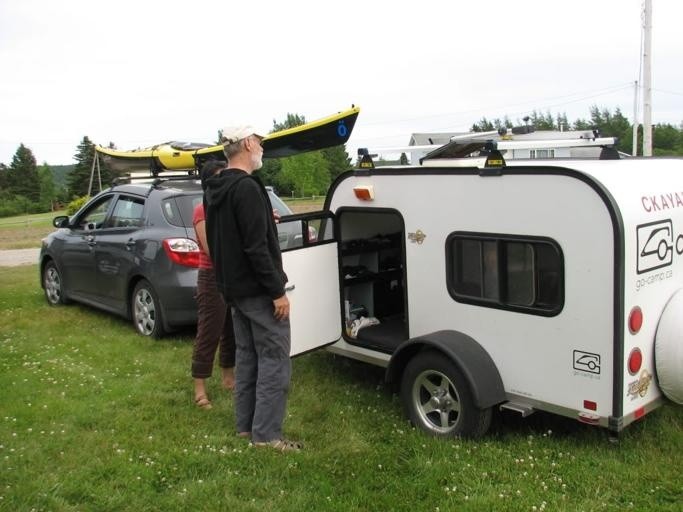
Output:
[202,122,306,453]
[191,160,236,409]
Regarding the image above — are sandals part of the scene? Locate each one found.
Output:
[194,391,212,412]
[237,429,303,454]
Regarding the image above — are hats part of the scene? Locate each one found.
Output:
[220,121,265,146]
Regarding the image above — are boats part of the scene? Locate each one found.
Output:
[92,107,363,173]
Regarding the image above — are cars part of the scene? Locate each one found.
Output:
[37,177,321,343]
